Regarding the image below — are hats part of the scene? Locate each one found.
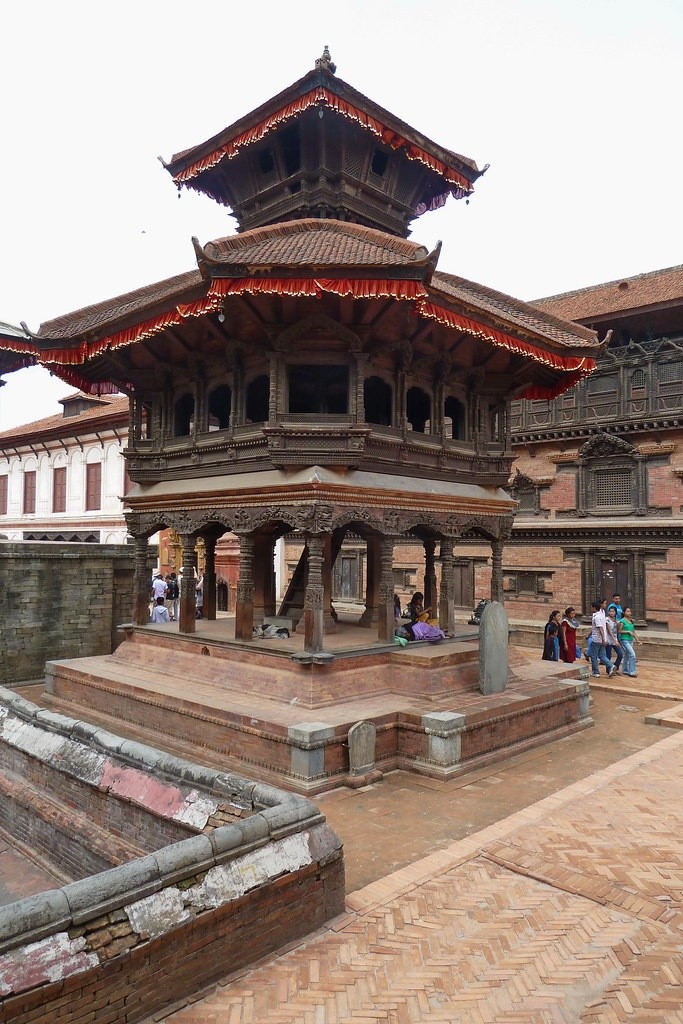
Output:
[179,566,182,573]
[153,568,161,577]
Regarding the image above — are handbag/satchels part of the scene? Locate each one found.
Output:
[575,645,580,658]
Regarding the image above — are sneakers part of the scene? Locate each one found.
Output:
[608,666,617,678]
[593,673,600,678]
[622,670,637,677]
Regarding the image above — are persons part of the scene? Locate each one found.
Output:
[394,594,410,618]
[607,594,622,622]
[193,567,204,613]
[606,606,623,676]
[584,598,617,678]
[559,607,578,663]
[151,597,170,623]
[411,592,438,627]
[542,610,560,662]
[617,607,642,677]
[151,566,184,621]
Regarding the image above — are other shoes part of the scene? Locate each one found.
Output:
[584,653,590,662]
